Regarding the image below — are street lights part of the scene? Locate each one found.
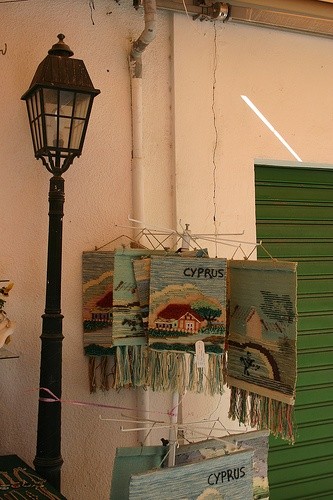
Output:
[18,34,101,497]
